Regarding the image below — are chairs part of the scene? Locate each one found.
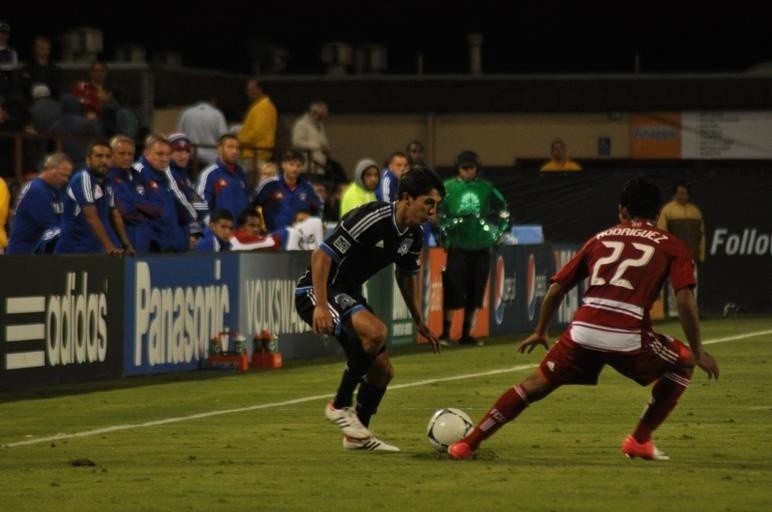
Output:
[271,128,289,165]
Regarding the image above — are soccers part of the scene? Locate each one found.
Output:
[428,408,472,451]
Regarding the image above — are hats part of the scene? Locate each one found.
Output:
[166,126,191,154]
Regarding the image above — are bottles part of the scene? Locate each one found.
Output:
[207,327,284,371]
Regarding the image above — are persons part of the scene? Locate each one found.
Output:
[434,151,511,346]
[447,177,720,460]
[656,185,706,264]
[540,141,582,171]
[295,167,445,451]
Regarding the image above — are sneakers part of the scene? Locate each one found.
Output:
[621,434,669,461]
[323,398,370,439]
[343,435,401,453]
[435,334,486,347]
[444,442,477,460]
